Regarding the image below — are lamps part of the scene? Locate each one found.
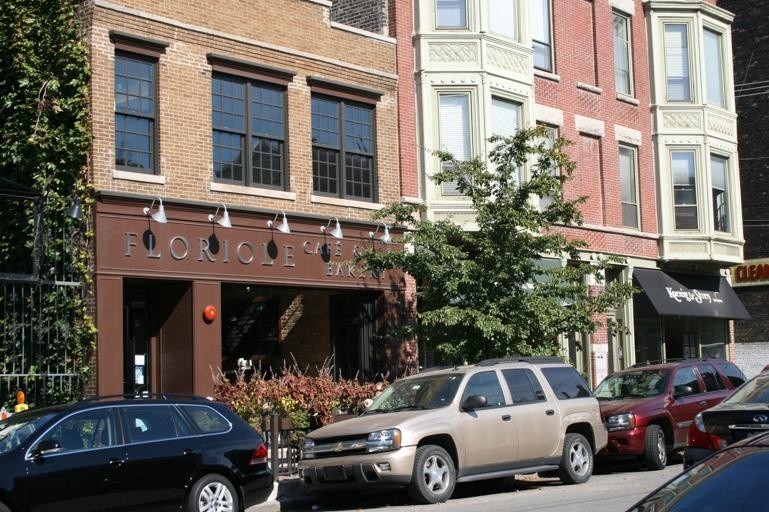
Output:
[143,195,393,243]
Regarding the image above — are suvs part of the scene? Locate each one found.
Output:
[0,390,275,511]
[297,353,614,505]
[580,357,748,470]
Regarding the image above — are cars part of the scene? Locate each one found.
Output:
[618,365,768,511]
